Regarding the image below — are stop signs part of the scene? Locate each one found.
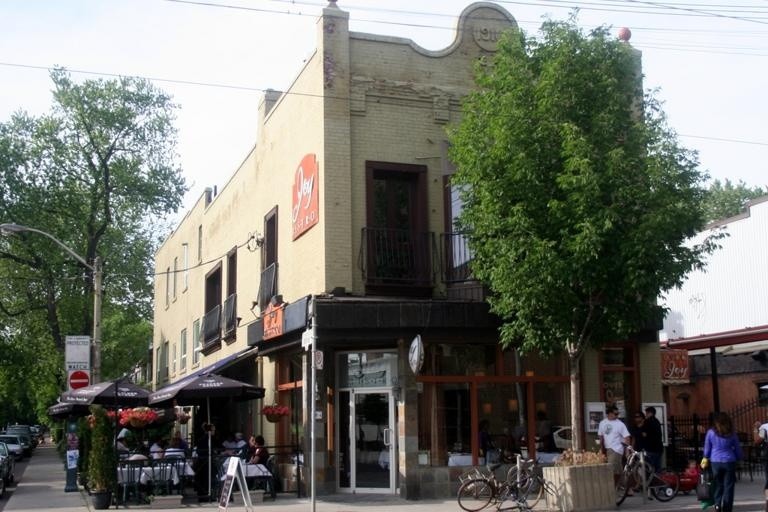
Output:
[67,369,90,390]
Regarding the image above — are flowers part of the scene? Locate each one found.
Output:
[259,403,290,417]
[107,404,190,430]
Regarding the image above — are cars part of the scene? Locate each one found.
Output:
[0,441,16,500]
[469,430,528,464]
[0,422,41,461]
[538,425,572,453]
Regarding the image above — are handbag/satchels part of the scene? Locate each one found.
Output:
[696,469,715,510]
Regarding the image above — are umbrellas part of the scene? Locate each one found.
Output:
[49,402,91,419]
[148,371,265,503]
[59,376,154,451]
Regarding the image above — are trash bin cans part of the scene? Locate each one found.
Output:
[56,428,63,445]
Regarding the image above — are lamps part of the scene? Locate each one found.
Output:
[247,300,289,319]
[235,315,261,328]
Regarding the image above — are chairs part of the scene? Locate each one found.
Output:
[116,442,271,506]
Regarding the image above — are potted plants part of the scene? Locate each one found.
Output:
[83,404,114,511]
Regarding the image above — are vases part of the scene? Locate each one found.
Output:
[266,414,280,423]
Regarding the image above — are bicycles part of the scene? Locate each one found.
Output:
[457,455,548,512]
[613,442,680,505]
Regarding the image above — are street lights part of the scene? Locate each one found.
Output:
[1,222,102,384]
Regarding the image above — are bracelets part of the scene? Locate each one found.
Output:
[753,430,758,433]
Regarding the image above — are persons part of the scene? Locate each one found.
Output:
[597,405,663,496]
[116,428,270,490]
[753,420,768,511]
[700,411,743,512]
